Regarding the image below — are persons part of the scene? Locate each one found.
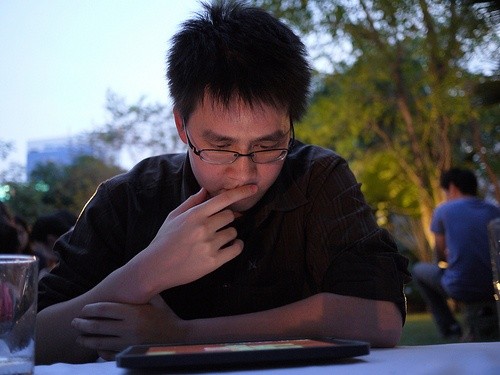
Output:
[35,0,414,366]
[0,201,79,326]
[410,168,500,342]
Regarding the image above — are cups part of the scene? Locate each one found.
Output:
[0,254,39,375]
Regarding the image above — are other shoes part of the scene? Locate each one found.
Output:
[429,301,463,340]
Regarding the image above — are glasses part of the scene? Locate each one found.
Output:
[183,121,295,165]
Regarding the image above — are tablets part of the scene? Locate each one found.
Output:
[116,336,370,368]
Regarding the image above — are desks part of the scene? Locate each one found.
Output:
[33,343,500,375]
